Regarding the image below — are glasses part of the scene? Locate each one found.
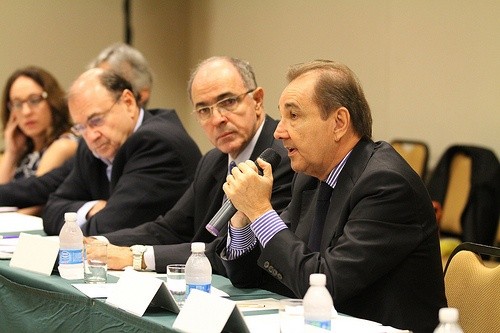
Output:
[9,94,47,112]
[70,97,121,136]
[191,90,252,120]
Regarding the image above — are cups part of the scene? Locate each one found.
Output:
[278,298,303,333]
[167,263,186,310]
[82,241,108,285]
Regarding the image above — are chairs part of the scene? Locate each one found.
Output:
[389,139,500,333]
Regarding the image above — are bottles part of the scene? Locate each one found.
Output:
[185,241,212,301]
[302,273,333,331]
[58,213,84,280]
[432,307,464,333]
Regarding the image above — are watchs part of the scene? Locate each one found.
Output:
[129,243,146,270]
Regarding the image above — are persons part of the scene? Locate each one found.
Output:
[0,42,152,217]
[42,68,205,247]
[213,58,451,333]
[68,55,302,301]
[0,67,84,221]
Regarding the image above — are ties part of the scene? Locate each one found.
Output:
[308,182,334,253]
[229,161,236,175]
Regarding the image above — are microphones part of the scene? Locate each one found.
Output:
[205,148,282,236]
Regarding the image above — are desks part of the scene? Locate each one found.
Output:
[0,203,379,333]
